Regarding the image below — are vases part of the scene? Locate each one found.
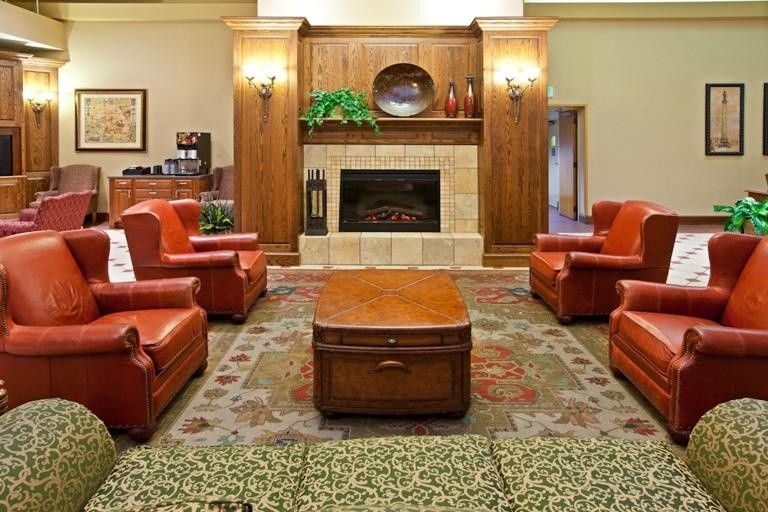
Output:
[445,80,458,118]
[463,72,477,118]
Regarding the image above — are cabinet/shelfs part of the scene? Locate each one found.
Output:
[0,175,27,220]
[105,171,215,229]
[21,170,54,210]
[0,58,24,128]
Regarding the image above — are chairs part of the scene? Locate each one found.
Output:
[28,163,102,227]
[526,198,680,326]
[0,227,213,446]
[602,230,768,447]
[197,164,237,223]
[0,189,94,240]
[119,197,271,326]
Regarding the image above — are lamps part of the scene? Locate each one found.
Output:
[502,68,540,125]
[244,71,279,123]
[23,90,54,129]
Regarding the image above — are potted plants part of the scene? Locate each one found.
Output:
[298,86,384,142]
[711,194,768,239]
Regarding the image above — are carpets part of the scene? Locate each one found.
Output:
[111,264,691,458]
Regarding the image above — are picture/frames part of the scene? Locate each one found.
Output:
[761,82,768,156]
[704,82,745,156]
[74,88,148,153]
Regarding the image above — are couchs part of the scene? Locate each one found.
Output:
[0,394,768,512]
